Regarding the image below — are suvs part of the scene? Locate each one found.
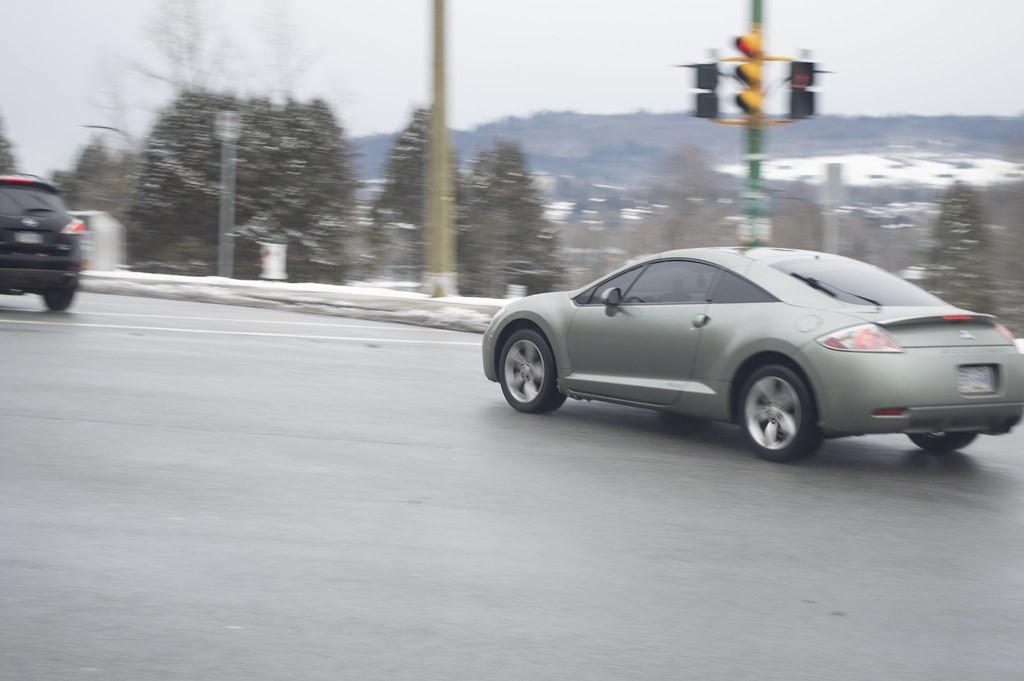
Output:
[0,171,88,312]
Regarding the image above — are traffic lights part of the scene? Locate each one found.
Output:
[682,62,721,123]
[734,24,762,113]
[789,60,817,117]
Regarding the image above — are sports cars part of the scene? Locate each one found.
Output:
[482,246,1024,465]
